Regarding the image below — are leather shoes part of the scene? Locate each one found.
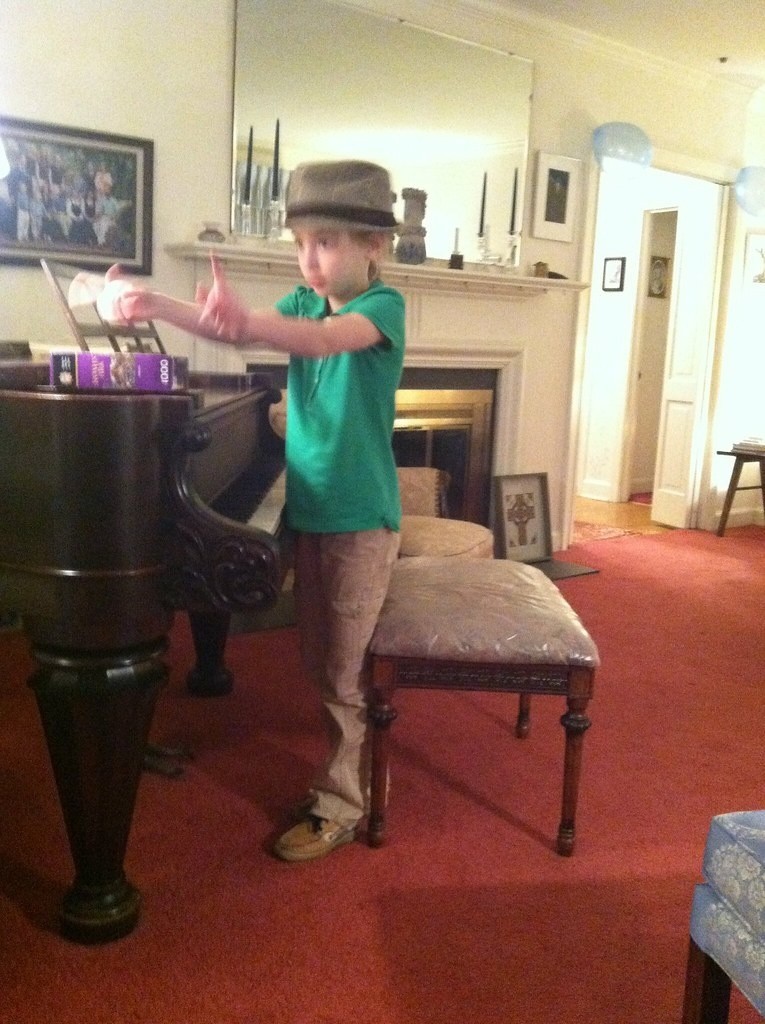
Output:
[277,816,356,861]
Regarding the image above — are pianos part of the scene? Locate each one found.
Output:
[0,259,292,938]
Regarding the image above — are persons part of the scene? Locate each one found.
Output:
[88,160,412,864]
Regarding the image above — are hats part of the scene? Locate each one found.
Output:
[287,161,401,231]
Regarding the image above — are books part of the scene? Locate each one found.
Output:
[48,347,192,393]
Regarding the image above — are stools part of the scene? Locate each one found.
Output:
[679,812,763,1023]
[362,554,599,857]
[714,450,765,537]
[393,512,497,558]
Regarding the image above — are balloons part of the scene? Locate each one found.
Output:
[591,120,655,175]
[733,164,765,219]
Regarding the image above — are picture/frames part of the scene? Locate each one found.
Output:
[602,257,626,293]
[647,255,669,299]
[530,150,584,242]
[494,470,554,566]
[0,115,155,278]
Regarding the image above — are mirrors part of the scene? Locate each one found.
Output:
[227,2,534,266]
